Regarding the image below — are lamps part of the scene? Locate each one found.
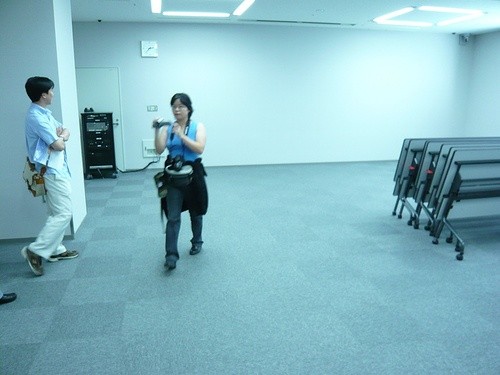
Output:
[372,6,487,27]
[151,0,256,17]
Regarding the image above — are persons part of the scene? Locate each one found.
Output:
[0,292,17,305]
[152,93,209,270]
[21,76,79,276]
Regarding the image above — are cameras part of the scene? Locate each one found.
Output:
[153,118,171,128]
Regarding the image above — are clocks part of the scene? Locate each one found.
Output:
[141,41,160,58]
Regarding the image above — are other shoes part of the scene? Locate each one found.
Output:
[189,244,201,255]
[0,293,17,305]
[164,259,176,269]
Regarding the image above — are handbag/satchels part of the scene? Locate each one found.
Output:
[22,158,47,198]
[154,172,164,198]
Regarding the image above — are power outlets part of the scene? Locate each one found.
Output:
[147,104,158,111]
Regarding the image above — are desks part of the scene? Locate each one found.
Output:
[392,137,500,261]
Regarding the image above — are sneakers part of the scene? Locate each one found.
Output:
[47,250,78,262]
[21,246,43,276]
